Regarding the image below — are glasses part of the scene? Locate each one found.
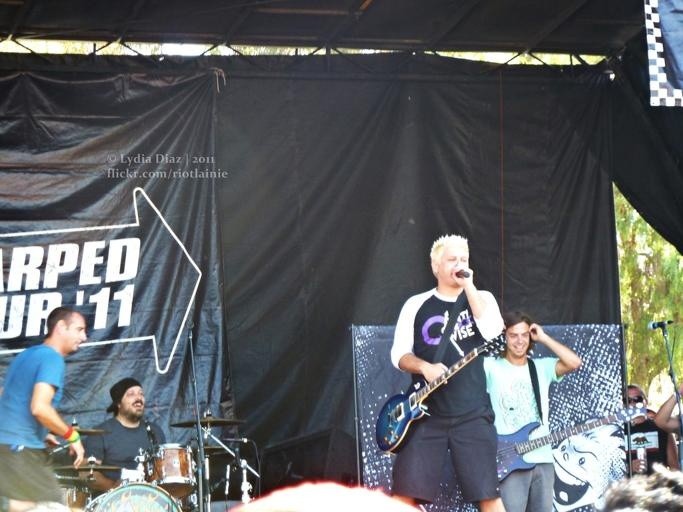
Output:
[622,393,646,403]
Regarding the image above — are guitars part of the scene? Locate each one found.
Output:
[376,335,507,452]
[497,401,647,484]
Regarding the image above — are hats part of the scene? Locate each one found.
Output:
[104,375,142,412]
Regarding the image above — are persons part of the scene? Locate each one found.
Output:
[621,386,679,480]
[598,461,682,512]
[652,378,682,442]
[389,233,507,511]
[481,309,581,512]
[0,306,86,511]
[77,377,165,501]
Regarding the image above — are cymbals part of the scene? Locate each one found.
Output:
[72,428,109,434]
[196,446,232,453]
[172,417,244,427]
[54,465,122,470]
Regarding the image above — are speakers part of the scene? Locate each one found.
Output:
[206,452,255,503]
[251,426,361,500]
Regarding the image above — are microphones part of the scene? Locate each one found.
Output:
[186,315,193,328]
[647,320,675,329]
[145,421,157,444]
[456,270,470,278]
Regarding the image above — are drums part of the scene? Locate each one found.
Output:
[135,443,197,496]
[87,481,184,512]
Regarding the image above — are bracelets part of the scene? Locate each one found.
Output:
[66,430,80,444]
[62,427,74,440]
[672,389,681,403]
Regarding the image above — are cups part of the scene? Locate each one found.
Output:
[636,445,647,473]
